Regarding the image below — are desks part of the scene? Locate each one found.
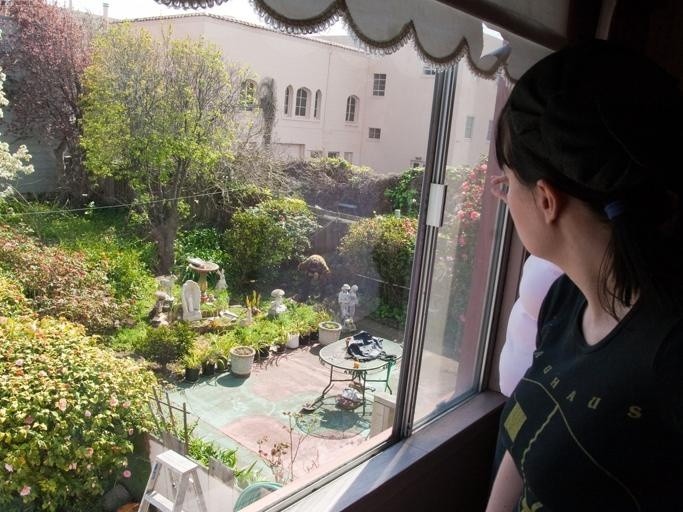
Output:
[319,335,402,417]
[189,261,220,302]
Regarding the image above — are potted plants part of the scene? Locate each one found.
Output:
[182,301,343,382]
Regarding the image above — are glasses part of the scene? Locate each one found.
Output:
[489,170,518,206]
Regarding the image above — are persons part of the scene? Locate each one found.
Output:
[481,39,682,512]
[338,284,359,324]
